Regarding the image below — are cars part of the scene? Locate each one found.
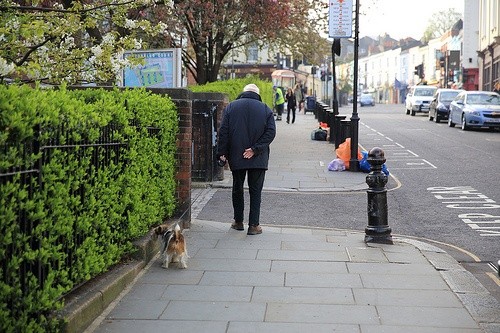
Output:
[348,95,360,103]
[360,94,375,107]
[428,87,467,123]
[448,90,500,132]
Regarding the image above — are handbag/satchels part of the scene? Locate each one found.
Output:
[311,127,328,141]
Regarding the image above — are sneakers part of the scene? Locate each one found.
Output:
[247,224,263,235]
[231,221,244,230]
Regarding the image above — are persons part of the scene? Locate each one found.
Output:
[493,82,500,94]
[219,84,276,235]
[286,88,296,123]
[293,84,305,111]
[273,86,285,120]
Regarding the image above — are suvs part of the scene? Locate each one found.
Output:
[404,85,438,116]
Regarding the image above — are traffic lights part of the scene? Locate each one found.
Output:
[440,56,446,68]
[327,71,331,81]
[320,71,325,81]
[415,63,424,78]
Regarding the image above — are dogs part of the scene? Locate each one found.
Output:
[148,222,190,270]
